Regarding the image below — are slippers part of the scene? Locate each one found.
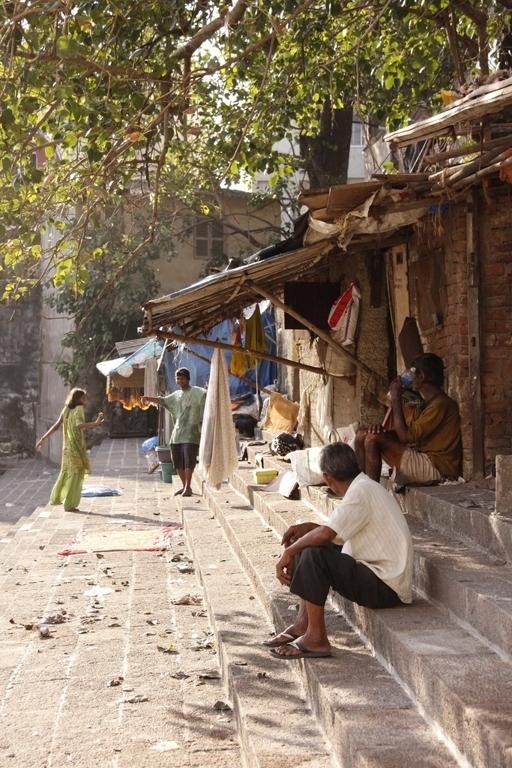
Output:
[268,642,332,659]
[262,631,300,646]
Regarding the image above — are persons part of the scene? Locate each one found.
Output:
[259,441,415,658]
[353,351,463,485]
[33,388,105,513]
[139,366,208,497]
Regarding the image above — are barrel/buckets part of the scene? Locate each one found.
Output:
[159,462,177,483]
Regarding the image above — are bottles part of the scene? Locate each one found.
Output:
[384,365,418,404]
